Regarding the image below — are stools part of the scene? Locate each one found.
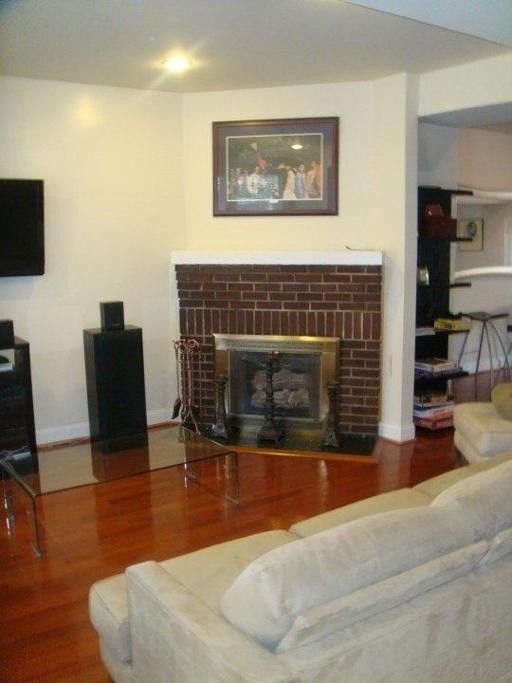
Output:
[458,311,511,392]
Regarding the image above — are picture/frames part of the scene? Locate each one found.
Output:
[212,116,340,217]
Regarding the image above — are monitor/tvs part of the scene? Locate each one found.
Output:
[0,179,44,276]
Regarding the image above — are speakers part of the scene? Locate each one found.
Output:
[100,301,124,332]
[0,323,15,349]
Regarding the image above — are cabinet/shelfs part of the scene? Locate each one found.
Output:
[414,186,474,434]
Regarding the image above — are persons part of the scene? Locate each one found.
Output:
[226,157,323,200]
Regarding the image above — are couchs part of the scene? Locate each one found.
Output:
[88,453,512,681]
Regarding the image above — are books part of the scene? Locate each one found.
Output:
[432,318,470,332]
[414,356,457,373]
[413,386,457,422]
[416,326,436,336]
